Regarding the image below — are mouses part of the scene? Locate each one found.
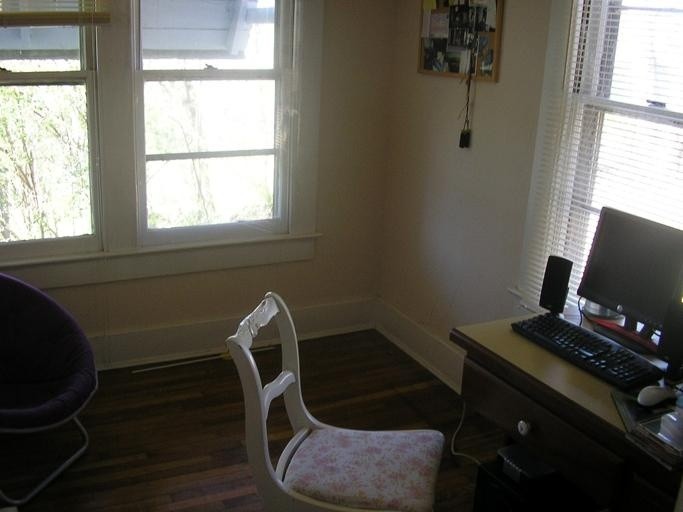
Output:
[637,385,675,406]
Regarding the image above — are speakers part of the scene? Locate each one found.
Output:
[657,301,683,380]
[540,255,573,319]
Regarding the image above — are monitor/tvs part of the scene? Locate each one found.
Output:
[577,207,683,354]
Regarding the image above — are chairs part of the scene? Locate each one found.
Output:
[0,273,99,506]
[226,293,446,512]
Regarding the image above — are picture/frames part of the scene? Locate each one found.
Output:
[417,0,503,82]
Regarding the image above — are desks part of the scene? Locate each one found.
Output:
[448,314,683,511]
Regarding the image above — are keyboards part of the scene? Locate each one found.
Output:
[511,314,663,392]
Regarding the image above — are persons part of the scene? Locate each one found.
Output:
[430,0,451,48]
[430,49,451,72]
[481,46,494,65]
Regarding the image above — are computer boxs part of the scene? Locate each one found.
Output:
[474,450,555,512]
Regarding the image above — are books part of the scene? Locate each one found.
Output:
[624,408,683,474]
